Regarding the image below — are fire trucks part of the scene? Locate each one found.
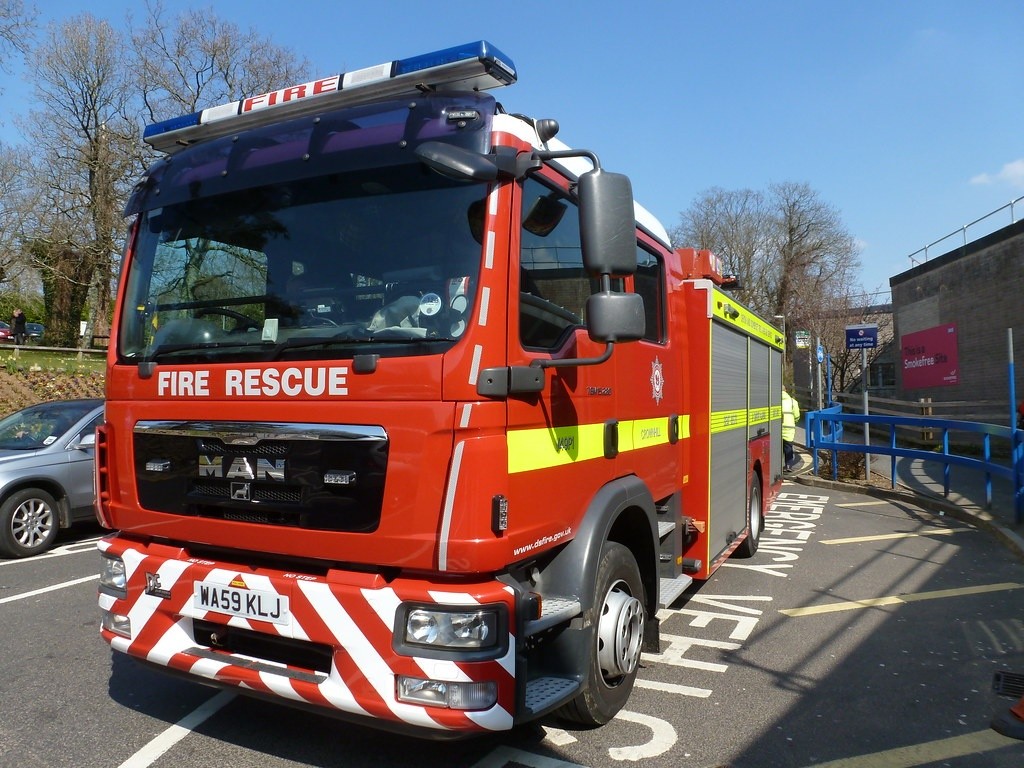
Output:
[92,41,787,745]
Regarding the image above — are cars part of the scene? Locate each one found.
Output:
[24,323,45,338]
[0,321,15,343]
[0,397,105,559]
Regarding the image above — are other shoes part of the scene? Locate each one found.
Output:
[786,465,792,472]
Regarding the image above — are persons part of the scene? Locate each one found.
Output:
[15,411,83,445]
[1015,411,1024,430]
[781,384,800,472]
[11,309,27,345]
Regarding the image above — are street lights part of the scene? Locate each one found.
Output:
[772,315,786,337]
